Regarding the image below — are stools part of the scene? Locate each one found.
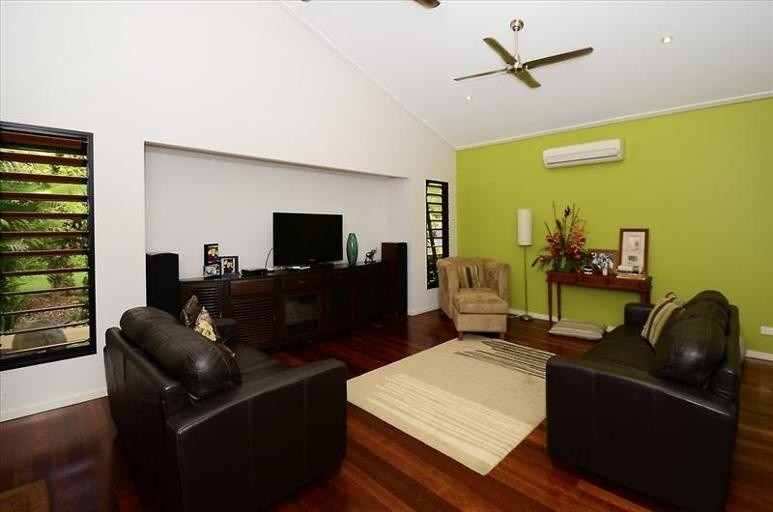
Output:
[450,290,508,342]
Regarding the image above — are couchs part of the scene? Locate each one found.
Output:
[543,290,746,512]
[100,306,350,511]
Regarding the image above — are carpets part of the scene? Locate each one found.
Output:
[341,333,560,476]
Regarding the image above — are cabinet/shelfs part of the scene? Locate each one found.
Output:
[179,279,224,321]
[355,260,387,330]
[276,271,321,349]
[224,275,277,351]
[321,267,354,339]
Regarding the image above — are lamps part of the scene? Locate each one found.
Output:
[515,207,534,323]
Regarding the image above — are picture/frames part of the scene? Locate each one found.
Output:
[203,242,220,266]
[615,226,649,273]
[202,261,220,280]
[219,256,239,279]
[585,247,620,277]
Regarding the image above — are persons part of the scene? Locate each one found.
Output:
[223,260,233,274]
[207,248,218,263]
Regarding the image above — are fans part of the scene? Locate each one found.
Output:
[450,18,594,91]
[300,0,441,10]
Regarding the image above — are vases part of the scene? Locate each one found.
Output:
[345,232,357,266]
[548,258,576,274]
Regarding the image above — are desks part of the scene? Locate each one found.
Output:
[543,267,653,334]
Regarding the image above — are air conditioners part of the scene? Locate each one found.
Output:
[541,138,624,170]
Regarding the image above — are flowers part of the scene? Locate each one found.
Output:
[529,202,593,275]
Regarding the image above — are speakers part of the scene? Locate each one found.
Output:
[382,242,407,320]
[146,254,178,319]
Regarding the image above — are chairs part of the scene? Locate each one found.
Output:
[433,253,510,329]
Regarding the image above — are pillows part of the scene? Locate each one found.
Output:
[191,306,237,362]
[181,295,203,330]
[665,291,685,309]
[454,264,482,290]
[637,297,686,351]
[545,318,608,340]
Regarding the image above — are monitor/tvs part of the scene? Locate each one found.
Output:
[273,212,343,272]
